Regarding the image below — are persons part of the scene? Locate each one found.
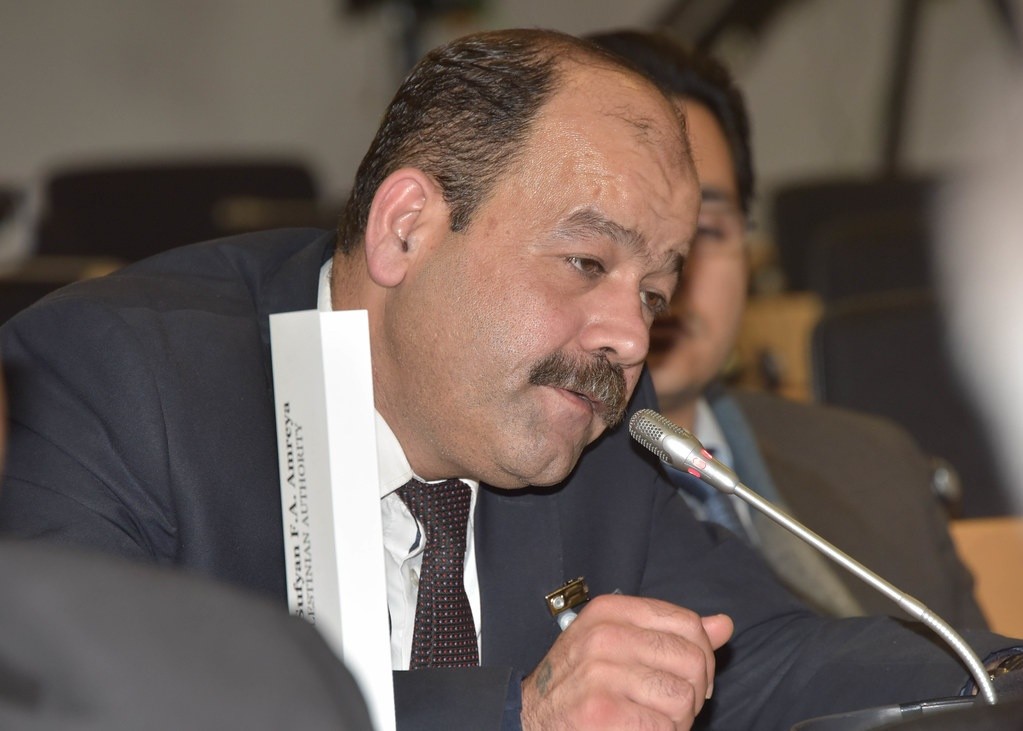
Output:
[576,24,994,638]
[1,27,1022,731]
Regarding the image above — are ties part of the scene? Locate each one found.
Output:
[395,478,479,670]
[678,449,747,545]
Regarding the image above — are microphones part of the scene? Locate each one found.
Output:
[627,407,1023,731]
